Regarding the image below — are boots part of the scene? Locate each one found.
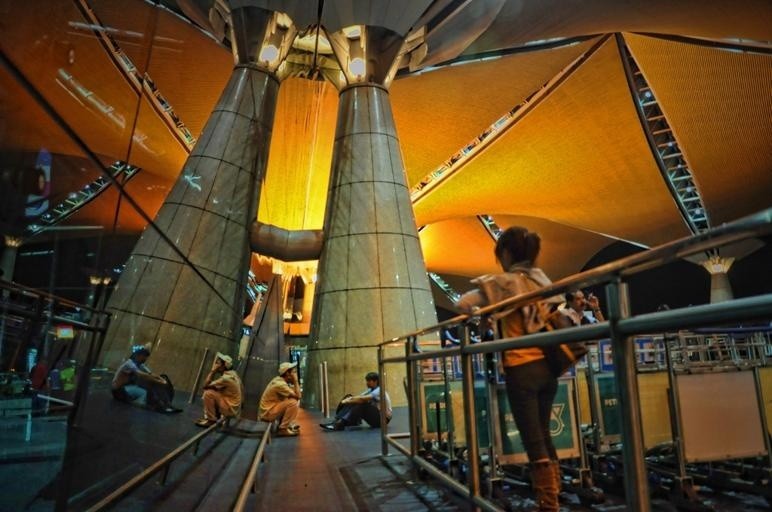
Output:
[528,459,561,512]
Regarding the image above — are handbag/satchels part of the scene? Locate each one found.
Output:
[536,308,589,371]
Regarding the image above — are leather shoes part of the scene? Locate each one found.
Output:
[194,418,214,426]
[319,422,345,430]
[277,428,299,437]
[158,406,184,413]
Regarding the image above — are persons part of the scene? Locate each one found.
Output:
[30,354,76,404]
[452,224,567,511]
[469,287,606,376]
[318,371,393,432]
[31,167,51,197]
[256,360,304,438]
[193,350,244,433]
[110,346,184,415]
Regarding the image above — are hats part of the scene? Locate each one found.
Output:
[216,351,233,369]
[279,362,298,376]
